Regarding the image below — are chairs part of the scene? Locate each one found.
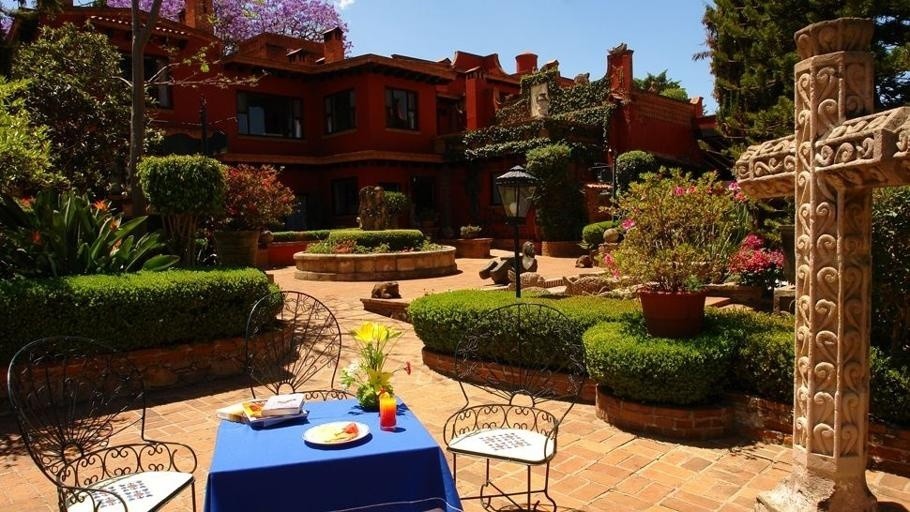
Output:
[453,303,587,511]
[243,291,357,402]
[10,336,199,512]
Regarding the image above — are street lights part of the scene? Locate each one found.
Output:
[592,145,621,229]
[495,165,540,298]
[198,94,229,160]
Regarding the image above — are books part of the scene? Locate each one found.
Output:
[218,391,311,430]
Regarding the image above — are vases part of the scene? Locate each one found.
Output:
[214,229,261,268]
[638,282,706,338]
[358,385,396,413]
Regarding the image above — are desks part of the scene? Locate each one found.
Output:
[204,391,465,512]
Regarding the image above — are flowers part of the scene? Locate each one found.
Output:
[337,317,412,410]
[595,166,788,294]
[206,160,305,232]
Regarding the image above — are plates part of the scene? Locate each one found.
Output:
[302,422,370,446]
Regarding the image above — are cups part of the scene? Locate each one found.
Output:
[380,396,397,431]
